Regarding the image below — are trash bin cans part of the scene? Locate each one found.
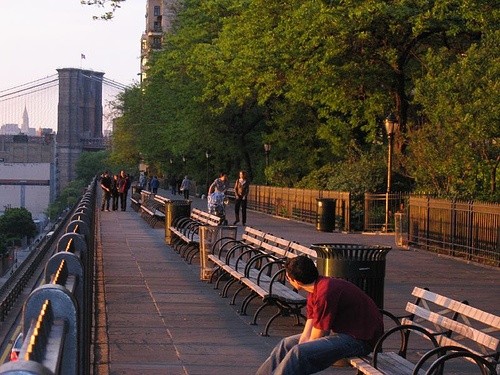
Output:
[164,200,193,243]
[132,186,144,195]
[311,243,392,366]
[315,197,337,232]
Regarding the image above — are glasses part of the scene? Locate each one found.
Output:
[285,276,290,282]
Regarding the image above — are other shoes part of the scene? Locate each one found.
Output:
[100,208,104,211]
[105,209,110,212]
[243,223,247,226]
[233,220,240,225]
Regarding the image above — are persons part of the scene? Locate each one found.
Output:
[181,176,189,199]
[257,256,384,375]
[177,176,182,195]
[208,172,228,225]
[100,170,131,211]
[170,176,176,194]
[233,171,249,225]
[136,174,158,193]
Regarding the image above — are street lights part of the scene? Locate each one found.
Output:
[264,141,272,186]
[206,148,212,197]
[384,112,400,233]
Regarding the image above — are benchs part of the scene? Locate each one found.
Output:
[129,190,318,339]
[344,285,500,375]
